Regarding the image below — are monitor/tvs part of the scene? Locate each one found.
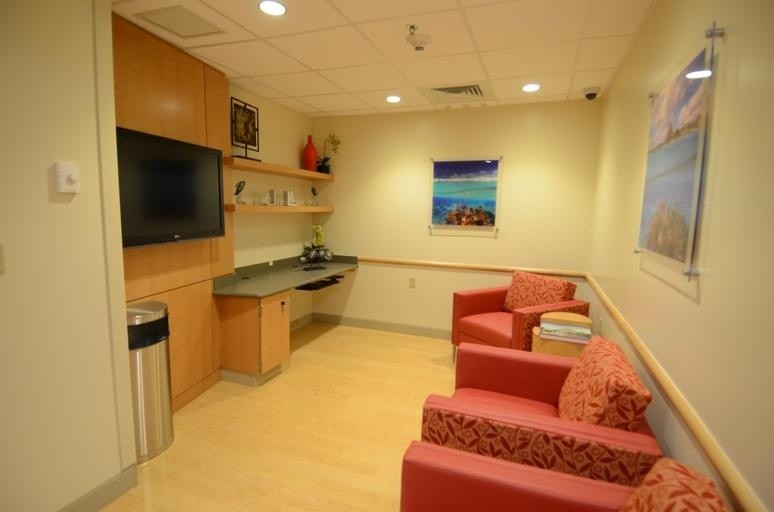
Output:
[116,126,225,248]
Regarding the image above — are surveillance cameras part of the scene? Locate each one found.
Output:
[583,87,600,101]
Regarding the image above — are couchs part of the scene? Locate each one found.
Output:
[401,439,635,511]
[452,285,591,362]
[423,341,664,486]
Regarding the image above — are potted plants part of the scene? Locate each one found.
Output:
[317,133,342,173]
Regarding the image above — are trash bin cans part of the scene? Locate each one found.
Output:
[127,301,173,466]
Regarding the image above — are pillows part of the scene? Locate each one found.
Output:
[558,334,652,431]
[625,457,729,511]
[503,270,577,312]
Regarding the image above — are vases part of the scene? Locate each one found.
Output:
[303,135,317,171]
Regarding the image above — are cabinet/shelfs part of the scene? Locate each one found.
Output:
[212,256,358,386]
[222,156,334,213]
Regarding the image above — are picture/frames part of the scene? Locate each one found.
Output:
[231,95,262,162]
